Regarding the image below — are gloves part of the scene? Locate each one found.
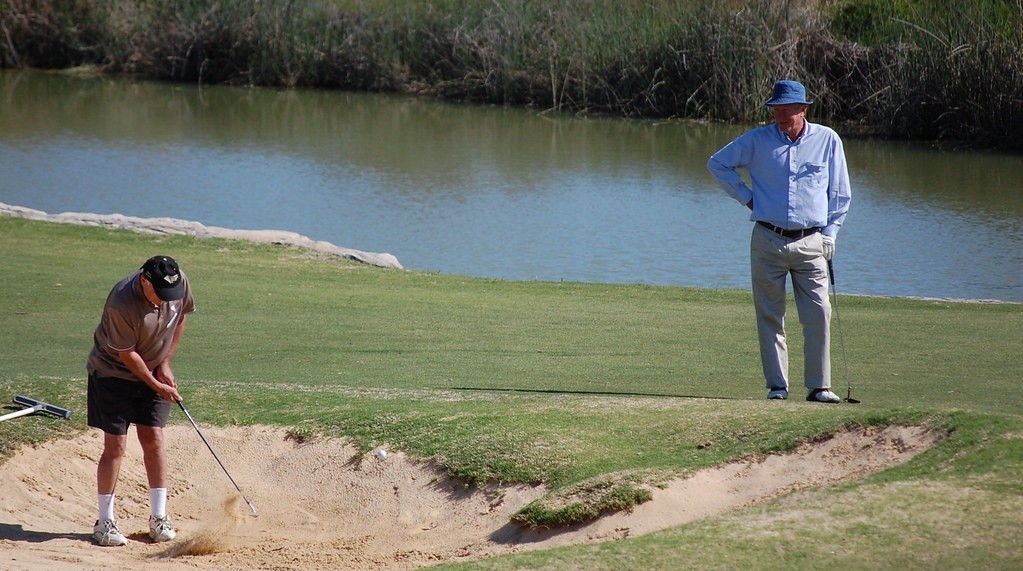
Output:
[822,236,835,260]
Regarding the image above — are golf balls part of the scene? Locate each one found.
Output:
[376,449,387,459]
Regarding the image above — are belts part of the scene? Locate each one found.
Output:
[757,221,822,238]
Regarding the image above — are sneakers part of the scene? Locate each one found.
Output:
[148,515,176,542]
[806,388,840,403]
[92,519,127,546]
[767,388,787,399]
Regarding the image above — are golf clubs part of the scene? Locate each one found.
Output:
[172,397,260,517]
[827,252,863,404]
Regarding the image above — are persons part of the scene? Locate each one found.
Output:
[706,81,852,402]
[86,252,198,547]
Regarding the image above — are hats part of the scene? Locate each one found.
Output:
[140,255,185,301]
[764,80,814,106]
